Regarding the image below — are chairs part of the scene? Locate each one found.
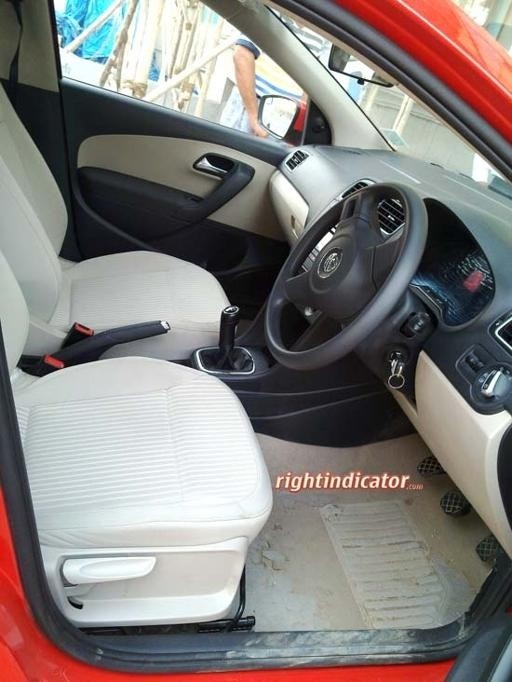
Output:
[0,252,273,627]
[0,82,237,360]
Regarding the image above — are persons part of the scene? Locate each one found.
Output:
[218,8,326,141]
